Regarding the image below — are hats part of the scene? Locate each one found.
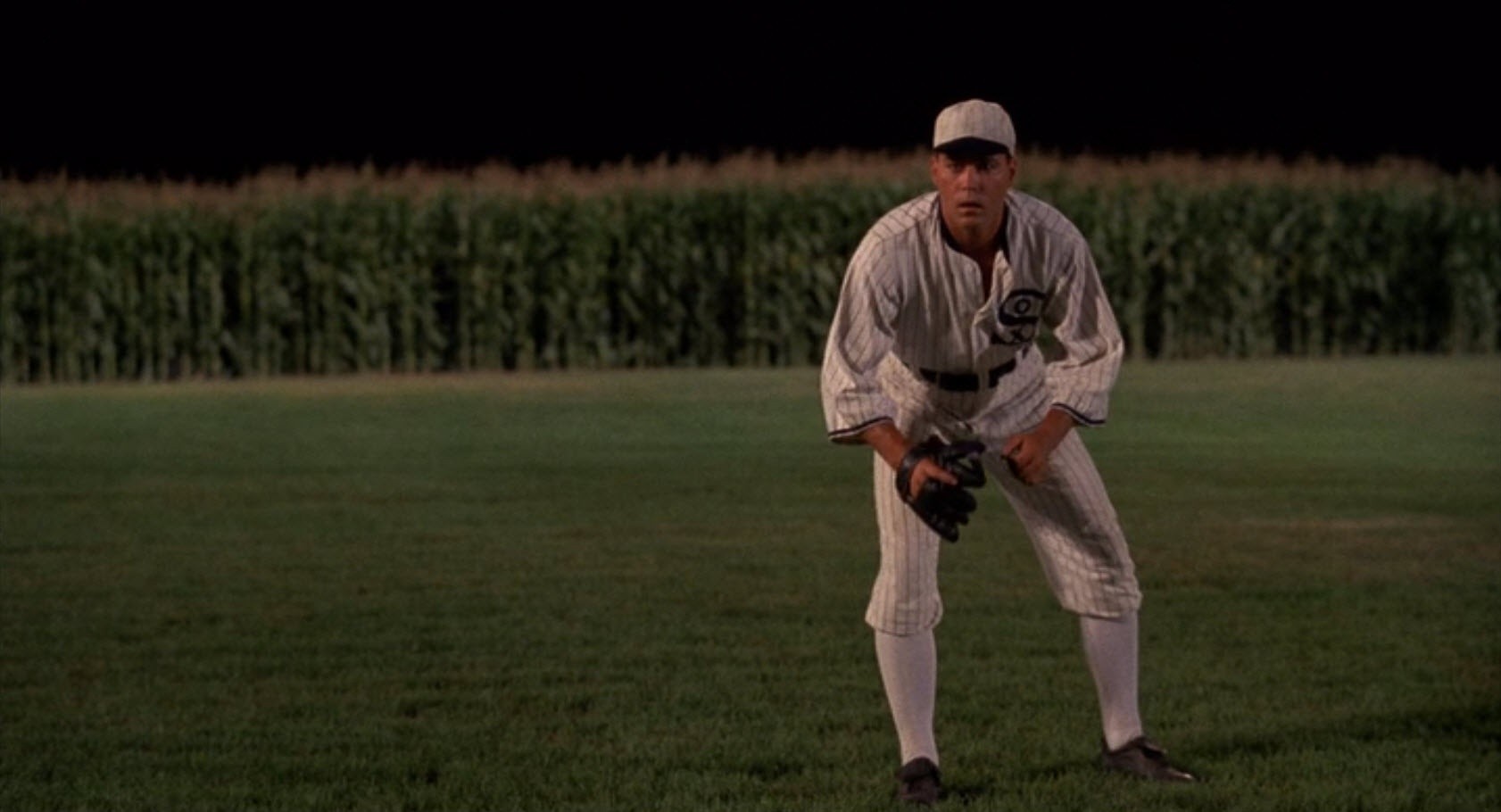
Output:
[932,99,1016,160]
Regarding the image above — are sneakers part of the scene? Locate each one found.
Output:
[896,755,941,806]
[1096,736,1204,784]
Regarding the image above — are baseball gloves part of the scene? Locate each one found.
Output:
[889,432,991,543]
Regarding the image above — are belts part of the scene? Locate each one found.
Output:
[902,359,1016,391]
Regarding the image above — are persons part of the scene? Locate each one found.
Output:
[820,98,1198,805]
